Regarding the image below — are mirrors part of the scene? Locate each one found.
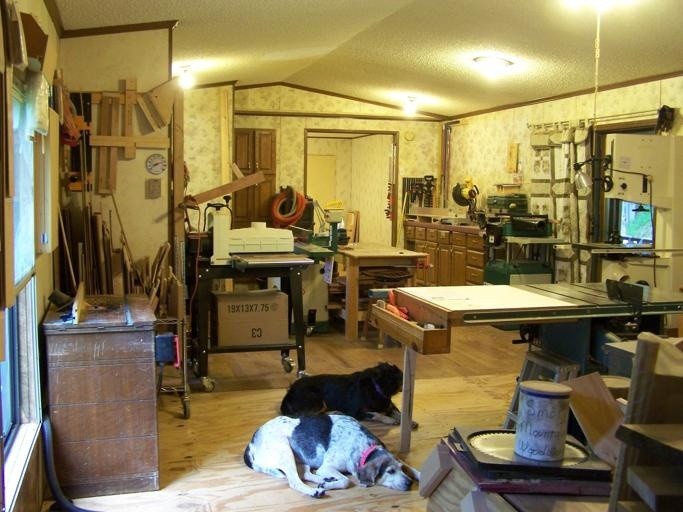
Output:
[304,129,399,247]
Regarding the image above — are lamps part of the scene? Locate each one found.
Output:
[574,155,612,190]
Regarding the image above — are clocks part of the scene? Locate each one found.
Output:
[145,153,167,175]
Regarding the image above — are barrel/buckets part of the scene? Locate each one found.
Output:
[514,379,572,462]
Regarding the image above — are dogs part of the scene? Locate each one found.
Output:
[245,414,421,498]
[280,363,419,430]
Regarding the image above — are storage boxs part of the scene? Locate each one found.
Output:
[209,288,289,348]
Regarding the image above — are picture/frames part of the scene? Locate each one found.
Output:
[2,72,36,309]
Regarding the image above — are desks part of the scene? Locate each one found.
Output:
[368,283,683,452]
[327,243,431,343]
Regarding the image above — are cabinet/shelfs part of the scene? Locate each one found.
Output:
[403,221,488,286]
[232,128,276,229]
[40,294,160,501]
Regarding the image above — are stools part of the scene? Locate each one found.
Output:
[360,270,413,349]
